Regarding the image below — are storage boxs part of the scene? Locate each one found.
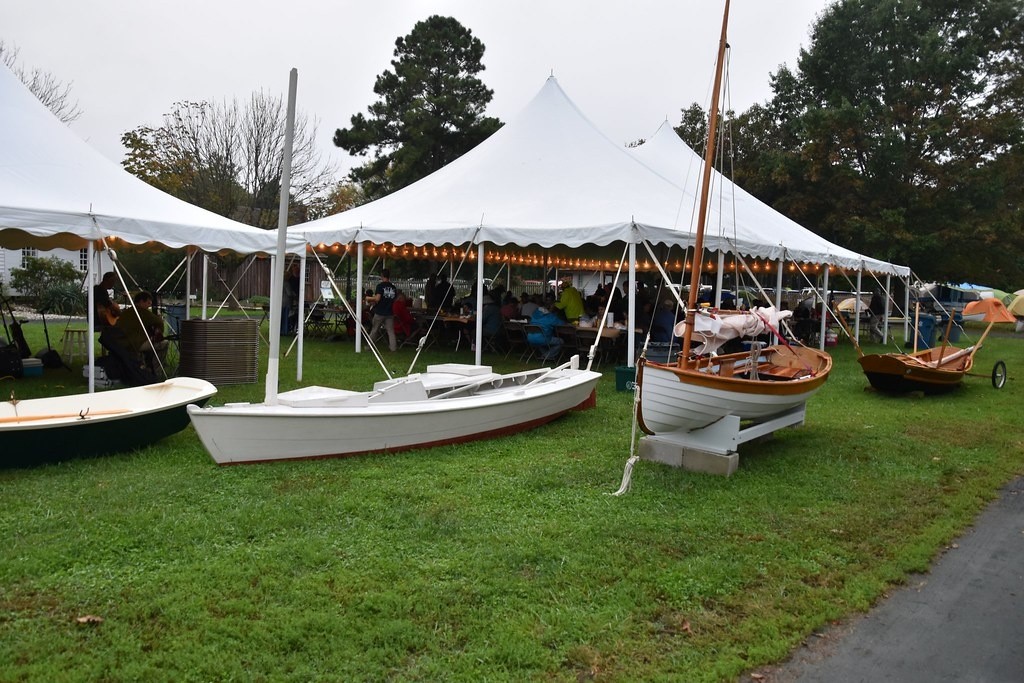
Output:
[23,364,43,376]
[615,367,636,393]
[741,341,767,351]
[826,334,838,347]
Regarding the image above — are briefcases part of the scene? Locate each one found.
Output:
[94,379,115,388]
[83,365,108,380]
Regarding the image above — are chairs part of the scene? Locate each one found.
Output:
[304,307,346,341]
[483,317,613,372]
[100,327,139,381]
[262,305,269,322]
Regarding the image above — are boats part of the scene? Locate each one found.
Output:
[0,199,222,473]
[857,342,975,392]
[915,297,983,313]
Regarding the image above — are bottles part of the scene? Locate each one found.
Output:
[325,299,328,309]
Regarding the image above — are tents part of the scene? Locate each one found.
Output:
[962,297,1018,324]
[840,297,867,312]
[0,54,305,402]
[275,70,913,370]
[1008,290,1024,317]
[915,281,1015,313]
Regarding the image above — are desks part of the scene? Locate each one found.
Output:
[574,322,642,362]
[421,316,469,351]
[320,307,349,334]
[400,312,427,348]
[880,316,943,339]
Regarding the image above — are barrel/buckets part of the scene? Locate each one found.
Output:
[616,366,636,391]
[826,334,837,346]
[167,305,191,334]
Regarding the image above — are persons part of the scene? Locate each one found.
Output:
[625,279,813,344]
[98,292,168,376]
[365,269,398,352]
[551,282,583,322]
[392,294,412,337]
[345,289,373,328]
[526,301,565,362]
[86,271,120,327]
[426,272,548,352]
[585,281,623,328]
[280,270,299,335]
[865,288,885,344]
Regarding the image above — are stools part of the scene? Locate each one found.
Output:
[63,329,89,365]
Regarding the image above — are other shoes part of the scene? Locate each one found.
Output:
[880,337,883,344]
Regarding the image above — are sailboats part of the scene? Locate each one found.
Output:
[634,1,834,439]
[186,65,604,467]
